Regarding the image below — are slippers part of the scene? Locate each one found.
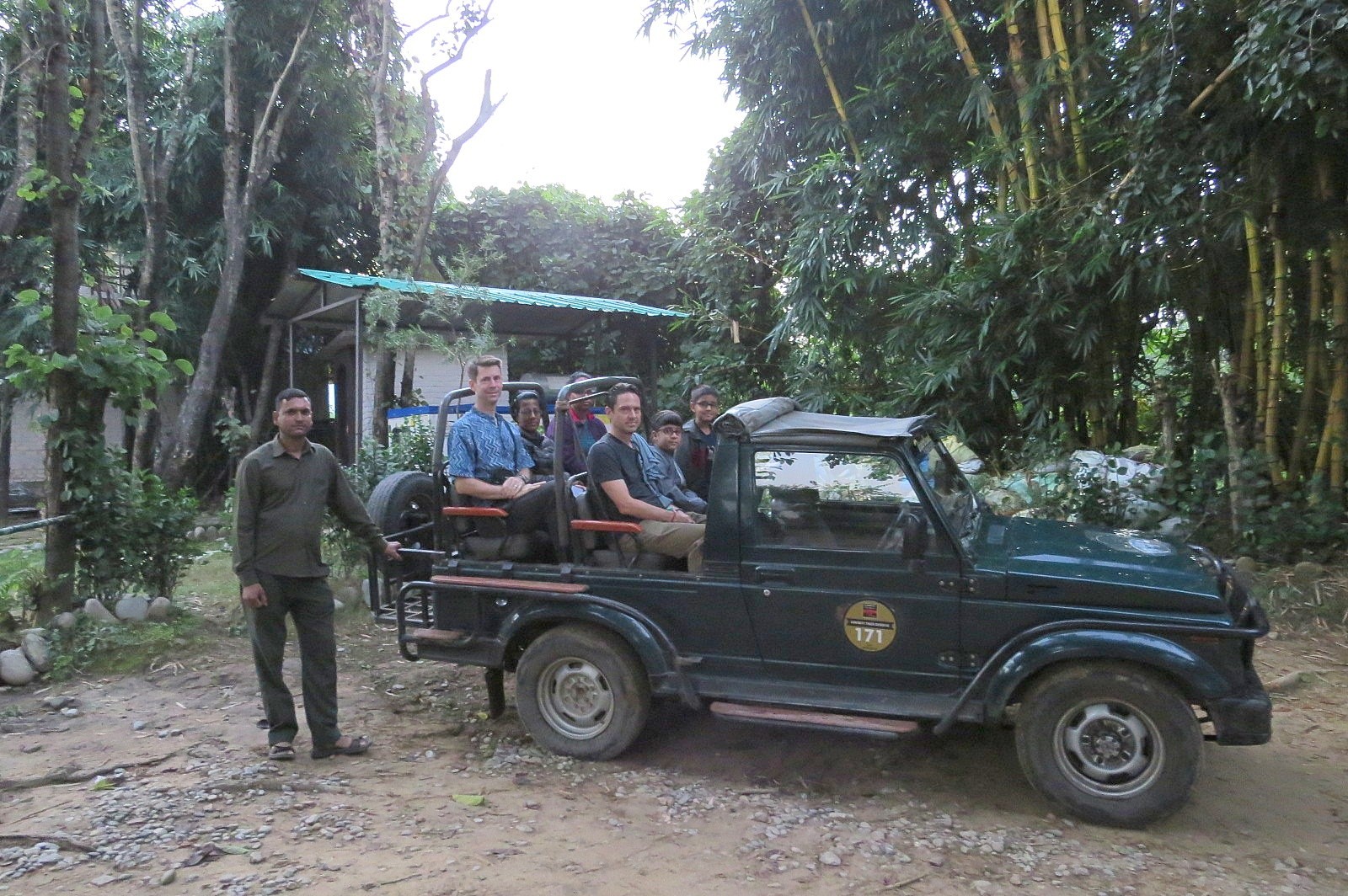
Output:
[310,734,373,758]
[269,743,295,759]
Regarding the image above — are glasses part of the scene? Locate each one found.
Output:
[656,426,682,437]
[574,389,597,399]
[695,401,718,409]
[517,407,542,416]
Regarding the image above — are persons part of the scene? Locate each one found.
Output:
[232,387,405,761]
[546,371,615,550]
[510,391,604,564]
[586,381,708,575]
[648,410,711,517]
[447,356,596,570]
[673,387,721,504]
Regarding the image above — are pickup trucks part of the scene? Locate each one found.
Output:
[361,375,1274,833]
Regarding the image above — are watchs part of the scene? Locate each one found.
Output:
[513,473,531,485]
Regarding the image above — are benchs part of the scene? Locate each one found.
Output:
[575,487,667,569]
[447,475,542,563]
